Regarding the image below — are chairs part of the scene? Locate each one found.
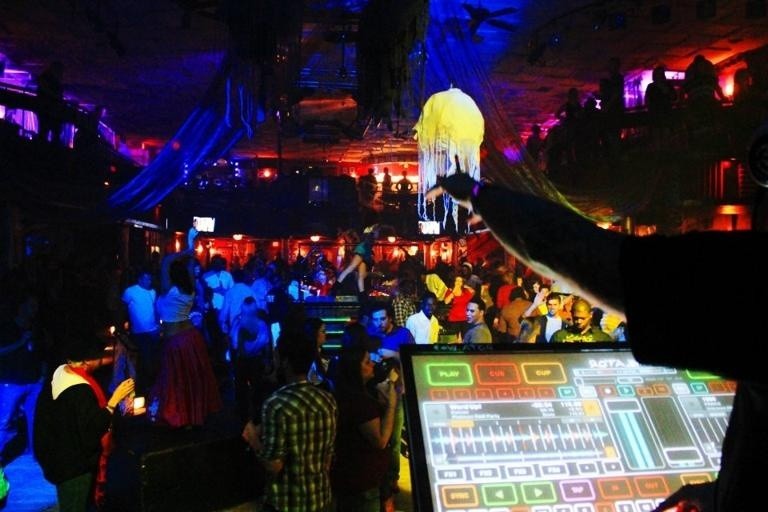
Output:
[125,439,239,511]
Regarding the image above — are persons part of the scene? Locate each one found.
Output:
[1,42,768,510]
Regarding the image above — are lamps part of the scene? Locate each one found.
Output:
[414,82,484,234]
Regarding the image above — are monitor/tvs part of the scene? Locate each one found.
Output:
[399,341,737,512]
